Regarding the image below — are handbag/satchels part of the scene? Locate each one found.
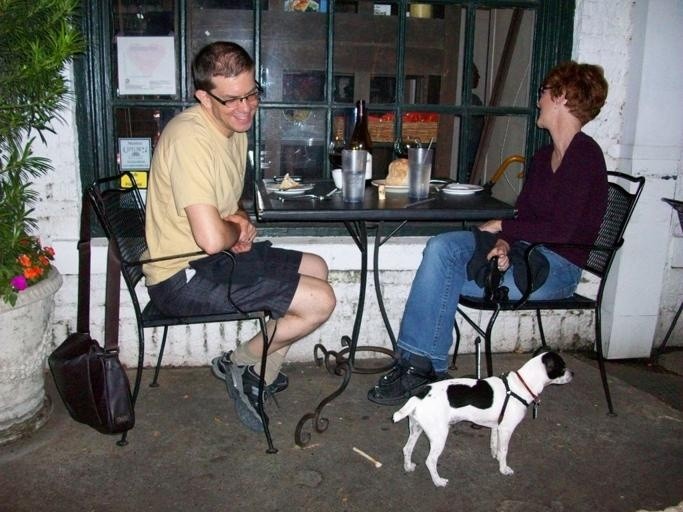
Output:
[48,332,136,435]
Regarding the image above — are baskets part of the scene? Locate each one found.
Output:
[331,114,439,145]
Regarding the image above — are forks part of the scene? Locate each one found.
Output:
[277,188,339,203]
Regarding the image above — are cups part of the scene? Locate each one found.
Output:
[408,4,432,19]
[394,136,422,159]
[407,147,433,200]
[340,150,366,204]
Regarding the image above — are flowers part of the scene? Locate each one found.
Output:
[0,0,99,307]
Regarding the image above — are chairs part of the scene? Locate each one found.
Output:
[88,170,280,454]
[653,198,682,364]
[449,170,645,429]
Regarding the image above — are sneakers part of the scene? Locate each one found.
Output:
[210,349,290,435]
[366,363,450,407]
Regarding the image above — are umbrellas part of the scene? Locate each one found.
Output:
[479,156,529,197]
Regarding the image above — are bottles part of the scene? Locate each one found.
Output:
[326,111,346,189]
[348,100,374,190]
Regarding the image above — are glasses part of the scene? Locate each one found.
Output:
[204,79,264,108]
[538,85,554,96]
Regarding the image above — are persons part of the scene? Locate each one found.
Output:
[464,61,485,185]
[141,42,337,435]
[368,61,609,406]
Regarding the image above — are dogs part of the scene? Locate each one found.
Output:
[390,345,575,488]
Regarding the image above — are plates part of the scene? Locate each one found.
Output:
[443,183,484,196]
[266,183,313,195]
[370,179,409,193]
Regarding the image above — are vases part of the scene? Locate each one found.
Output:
[0,265,63,449]
